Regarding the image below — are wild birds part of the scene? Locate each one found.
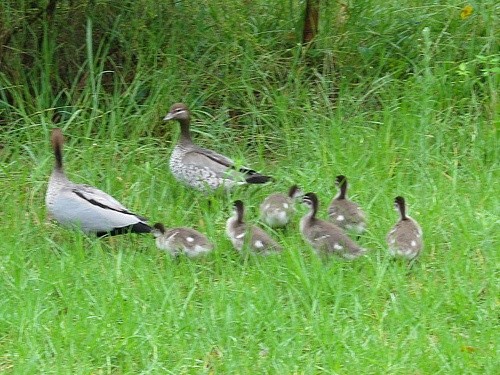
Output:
[326,175,368,242]
[162,101,275,214]
[384,196,423,272]
[259,184,305,237]
[44,128,154,241]
[225,199,285,258]
[151,223,214,264]
[298,192,366,265]
